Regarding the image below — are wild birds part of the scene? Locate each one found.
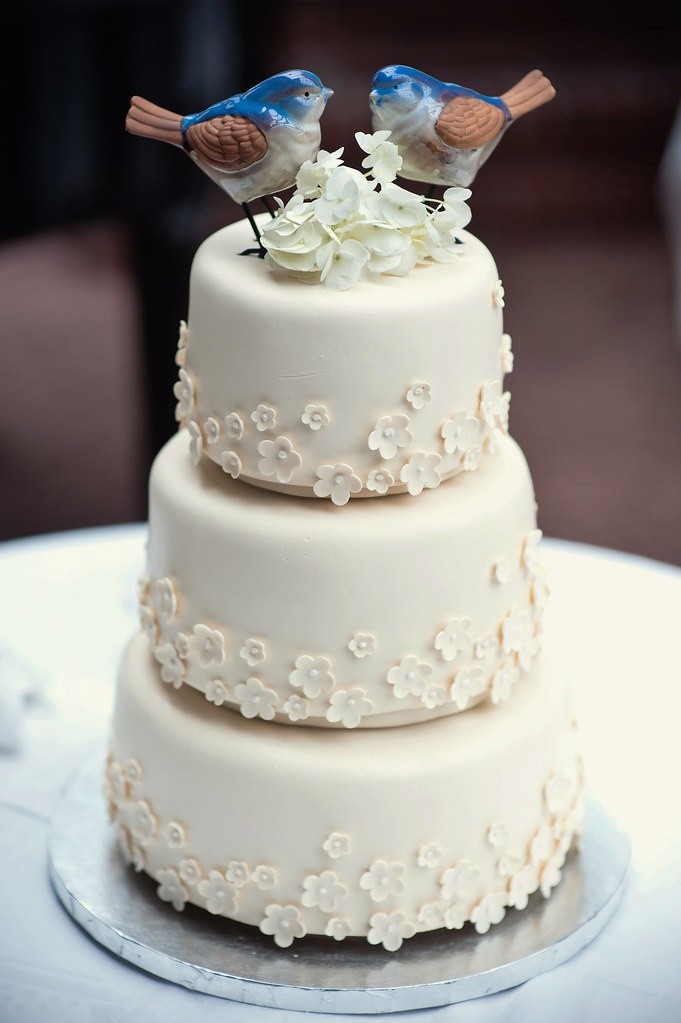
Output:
[367,64,557,244]
[123,68,336,260]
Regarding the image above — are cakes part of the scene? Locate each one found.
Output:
[101,62,581,952]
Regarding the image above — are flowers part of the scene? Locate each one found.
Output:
[259,128,473,293]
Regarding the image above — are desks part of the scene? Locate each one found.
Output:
[0,522,681,1023]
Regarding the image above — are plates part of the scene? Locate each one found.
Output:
[48,765,634,1015]
